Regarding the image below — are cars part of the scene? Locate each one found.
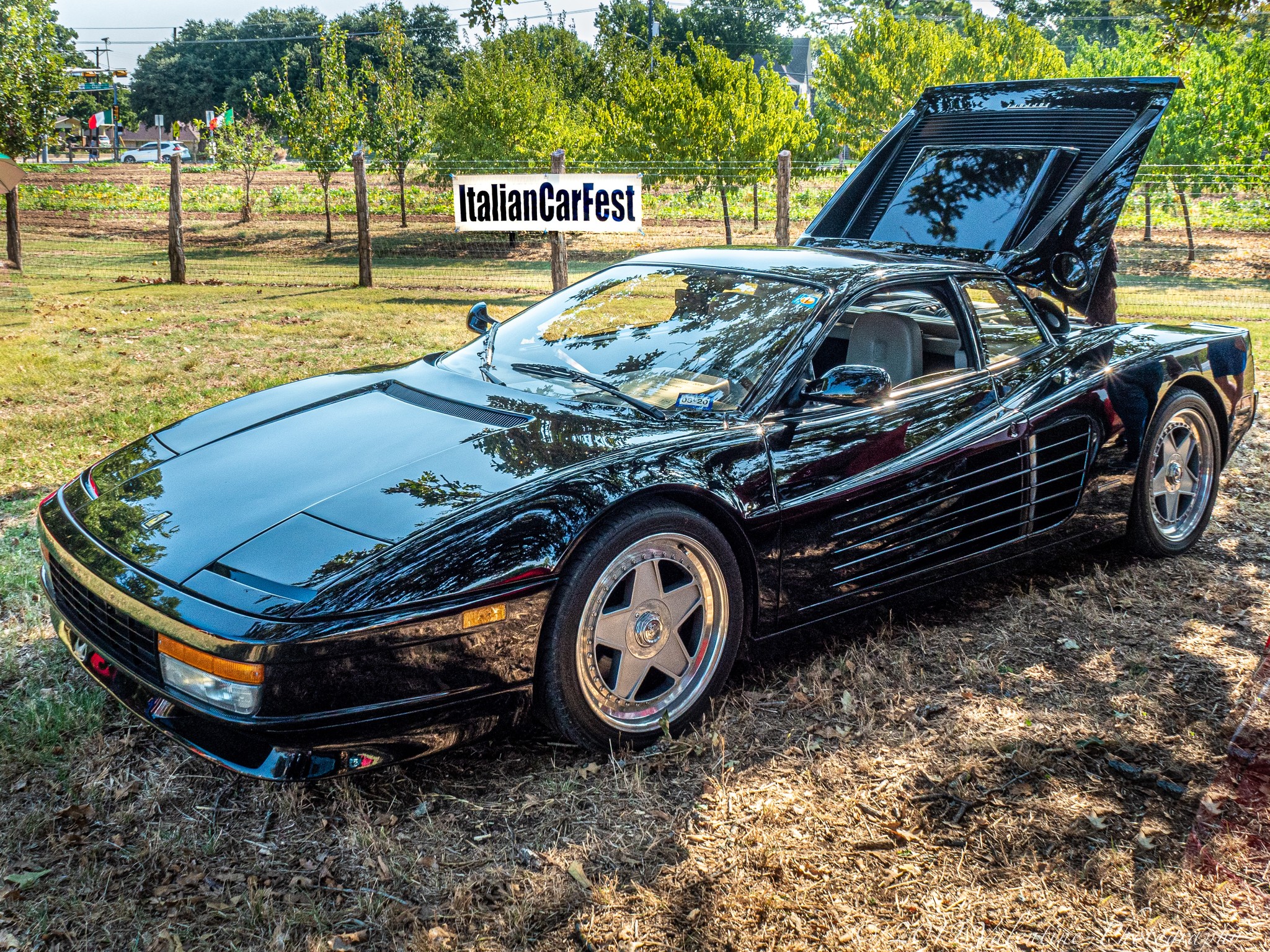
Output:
[91,135,110,145]
[37,75,1259,784]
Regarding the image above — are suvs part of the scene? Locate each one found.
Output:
[120,141,191,164]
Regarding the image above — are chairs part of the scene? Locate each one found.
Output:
[702,291,770,404]
[846,311,922,388]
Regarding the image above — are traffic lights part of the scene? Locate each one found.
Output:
[113,106,119,120]
